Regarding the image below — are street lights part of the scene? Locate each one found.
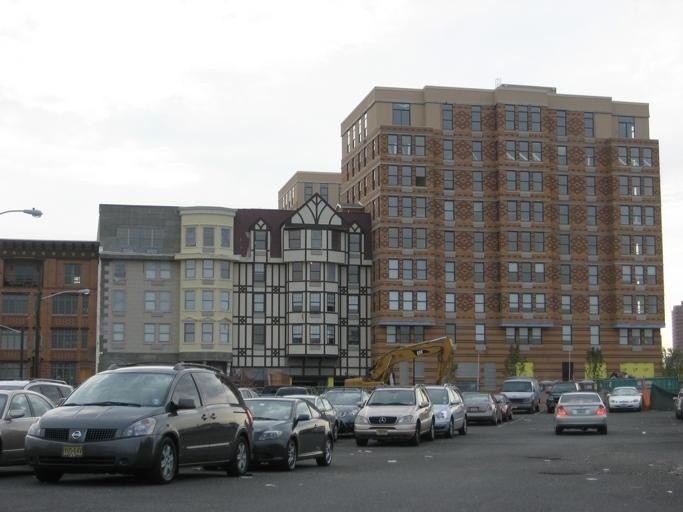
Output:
[32,288,91,378]
[475,344,487,390]
[563,344,574,380]
[0,208,42,218]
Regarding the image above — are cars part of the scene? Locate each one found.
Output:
[422,385,466,437]
[540,380,561,393]
[242,397,333,471]
[461,391,502,425]
[0,389,57,467]
[323,387,370,433]
[24,362,253,485]
[236,385,334,396]
[606,386,643,412]
[492,393,513,421]
[553,391,606,435]
[672,388,683,418]
[353,385,434,447]
[282,395,340,441]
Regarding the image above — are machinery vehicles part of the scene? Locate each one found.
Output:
[343,337,454,390]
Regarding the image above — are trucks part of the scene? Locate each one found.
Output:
[499,376,540,414]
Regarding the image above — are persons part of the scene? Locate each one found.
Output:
[609,369,617,379]
[620,370,629,379]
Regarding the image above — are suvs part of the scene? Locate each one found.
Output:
[0,378,73,407]
[545,381,582,412]
